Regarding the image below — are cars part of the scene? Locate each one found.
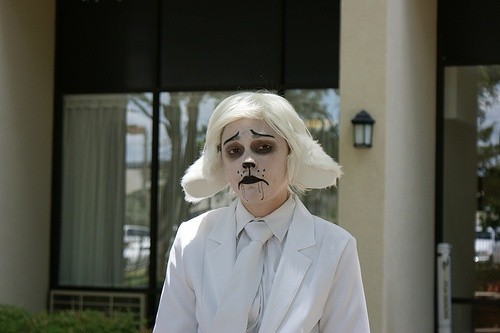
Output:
[123,225,153,269]
[475,224,500,266]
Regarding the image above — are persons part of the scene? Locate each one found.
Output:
[152,91,370,333]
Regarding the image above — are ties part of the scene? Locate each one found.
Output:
[212,222,274,333]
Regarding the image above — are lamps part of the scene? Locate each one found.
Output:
[351,110,375,147]
